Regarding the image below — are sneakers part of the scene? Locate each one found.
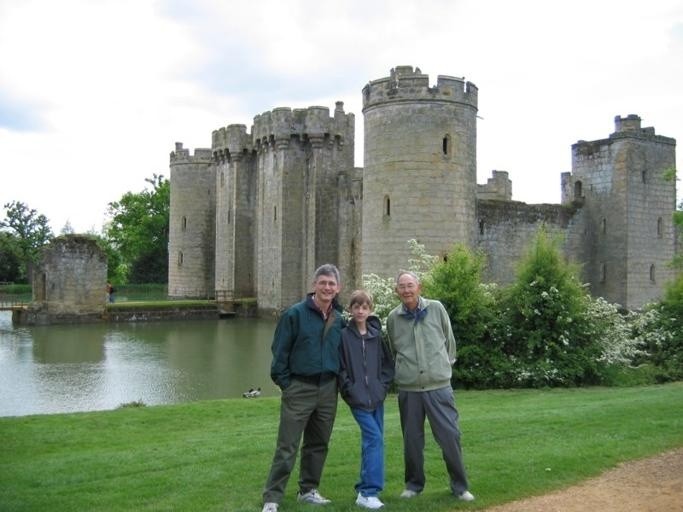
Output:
[261,501,279,512]
[295,490,332,504]
[400,490,418,498]
[355,491,384,509]
[458,490,474,501]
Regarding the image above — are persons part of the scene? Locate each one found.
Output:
[107,280,115,303]
